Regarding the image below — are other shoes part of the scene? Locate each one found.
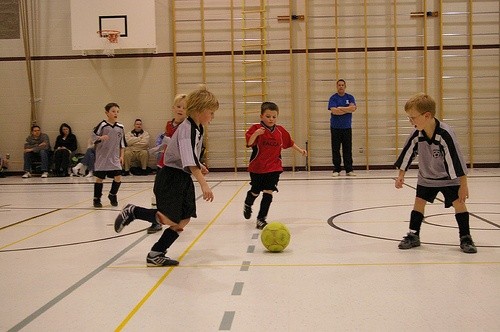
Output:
[92,197,102,207]
[108,194,118,206]
[22,171,32,178]
[398,230,421,249]
[41,171,48,177]
[243,201,253,219]
[347,171,357,176]
[331,172,341,176]
[147,223,162,234]
[256,218,267,228]
[460,234,478,253]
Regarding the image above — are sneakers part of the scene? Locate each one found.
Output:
[114,204,135,233]
[146,250,179,267]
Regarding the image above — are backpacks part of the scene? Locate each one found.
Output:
[71,153,89,175]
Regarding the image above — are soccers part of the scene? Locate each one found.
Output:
[260,222,290,253]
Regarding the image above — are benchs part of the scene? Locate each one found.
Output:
[30,161,151,175]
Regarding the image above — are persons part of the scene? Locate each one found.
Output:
[122,119,149,176]
[72,127,97,177]
[54,123,77,176]
[147,93,188,234]
[243,101,307,229]
[328,79,357,177]
[394,94,477,254]
[91,102,128,207]
[114,88,220,267]
[22,125,52,178]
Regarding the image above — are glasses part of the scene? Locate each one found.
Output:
[407,111,428,120]
